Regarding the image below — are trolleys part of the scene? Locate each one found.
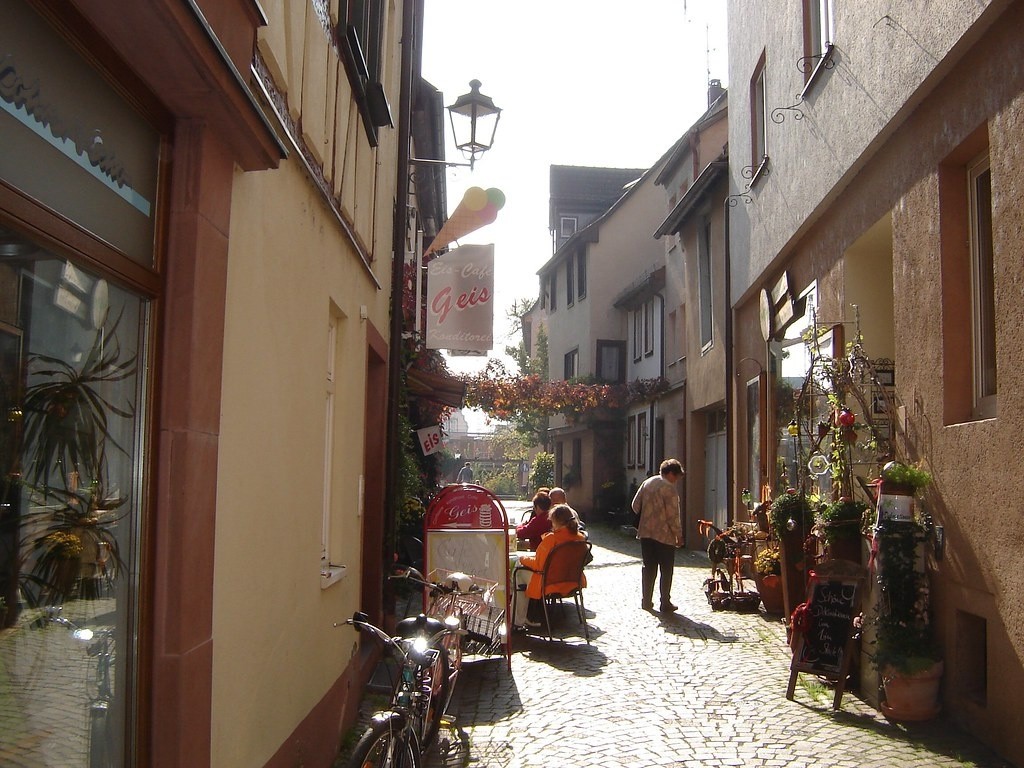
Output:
[698,519,741,612]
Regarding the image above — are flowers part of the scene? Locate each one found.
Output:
[848,583,944,690]
[753,546,814,576]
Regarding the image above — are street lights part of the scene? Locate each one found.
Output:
[381,77,502,656]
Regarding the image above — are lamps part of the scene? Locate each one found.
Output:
[409,78,504,172]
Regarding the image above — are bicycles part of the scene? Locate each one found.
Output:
[332,566,506,768]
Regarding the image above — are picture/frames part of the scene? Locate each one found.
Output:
[873,397,894,413]
[873,370,894,386]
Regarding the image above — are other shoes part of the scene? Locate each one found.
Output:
[642,602,654,609]
[523,617,542,626]
[660,604,678,612]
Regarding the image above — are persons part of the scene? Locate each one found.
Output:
[475,480,482,493]
[519,506,587,628]
[516,487,592,565]
[631,458,685,614]
[457,462,473,484]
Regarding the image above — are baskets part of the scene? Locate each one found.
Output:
[460,604,506,658]
[427,568,498,616]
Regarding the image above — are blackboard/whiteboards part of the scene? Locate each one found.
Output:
[421,484,512,646]
[790,575,858,681]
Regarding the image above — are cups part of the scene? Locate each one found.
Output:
[510,517,515,524]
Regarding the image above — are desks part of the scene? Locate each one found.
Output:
[509,551,536,628]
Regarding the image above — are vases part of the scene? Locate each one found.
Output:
[879,659,945,711]
[753,572,806,615]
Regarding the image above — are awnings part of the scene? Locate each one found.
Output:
[408,368,467,410]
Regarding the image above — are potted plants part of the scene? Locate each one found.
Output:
[752,321,933,584]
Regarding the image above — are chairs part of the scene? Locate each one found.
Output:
[511,540,593,647]
